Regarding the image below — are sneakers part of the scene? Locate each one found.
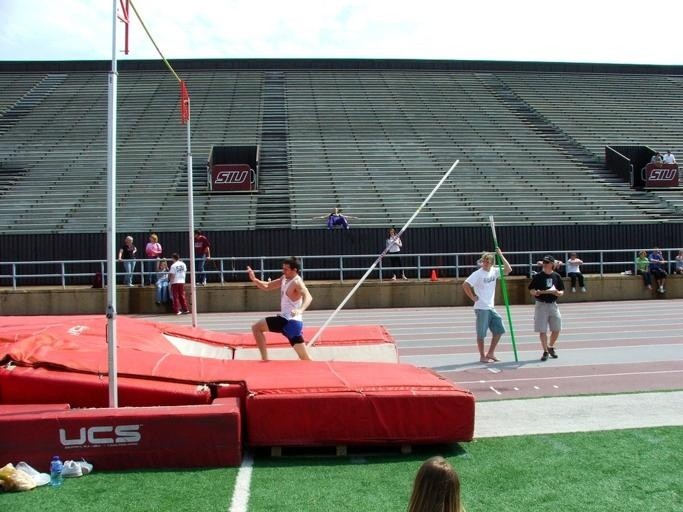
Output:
[548,346,558,358]
[541,352,549,361]
[402,275,407,280]
[16,462,51,486]
[61,461,83,477]
[392,276,396,280]
[64,461,93,474]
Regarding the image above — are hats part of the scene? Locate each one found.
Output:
[540,255,554,263]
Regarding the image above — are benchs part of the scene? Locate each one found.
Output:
[1,59,683,234]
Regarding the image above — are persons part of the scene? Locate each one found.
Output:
[245,257,314,361]
[637,247,683,293]
[118,229,211,315]
[650,152,662,163]
[528,255,565,361]
[314,209,358,231]
[406,456,464,512]
[463,248,513,363]
[567,253,586,293]
[385,228,407,280]
[663,150,676,164]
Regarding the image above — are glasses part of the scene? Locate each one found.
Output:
[543,261,549,264]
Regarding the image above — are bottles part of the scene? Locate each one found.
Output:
[50,455,63,489]
